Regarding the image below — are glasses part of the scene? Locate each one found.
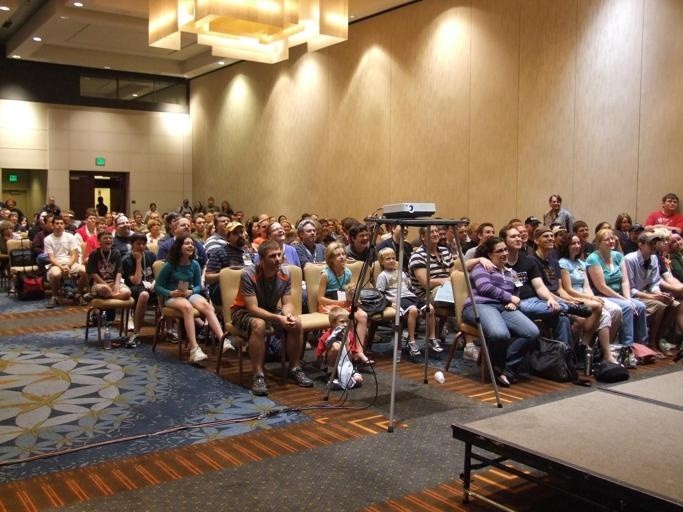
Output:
[492,248,509,254]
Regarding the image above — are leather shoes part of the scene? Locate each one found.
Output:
[496,375,511,386]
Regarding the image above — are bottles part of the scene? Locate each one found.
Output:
[100,311,108,327]
[103,327,111,350]
[584,347,594,377]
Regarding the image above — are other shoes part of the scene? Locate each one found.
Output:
[353,370,362,384]
[352,355,376,365]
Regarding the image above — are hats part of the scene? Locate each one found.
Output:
[11,250,34,266]
[533,226,552,251]
[552,225,567,241]
[629,223,644,231]
[257,214,274,225]
[525,216,541,226]
[225,221,245,237]
[638,232,661,244]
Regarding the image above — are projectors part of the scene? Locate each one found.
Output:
[381,203,437,220]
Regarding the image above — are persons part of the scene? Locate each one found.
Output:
[408,194,683,387]
[0,193,443,398]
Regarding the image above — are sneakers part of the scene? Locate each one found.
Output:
[250,374,268,396]
[462,345,479,361]
[659,338,676,351]
[188,347,208,363]
[287,367,313,387]
[568,302,592,318]
[427,338,443,352]
[222,337,235,354]
[46,286,134,329]
[165,330,180,343]
[407,341,421,357]
[126,333,141,348]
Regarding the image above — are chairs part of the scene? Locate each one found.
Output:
[7,238,34,297]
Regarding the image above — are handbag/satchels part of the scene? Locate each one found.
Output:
[523,335,658,386]
[359,288,387,314]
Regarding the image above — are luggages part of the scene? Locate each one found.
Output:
[18,238,46,301]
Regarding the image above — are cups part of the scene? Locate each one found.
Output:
[433,370,446,384]
[396,350,402,363]
